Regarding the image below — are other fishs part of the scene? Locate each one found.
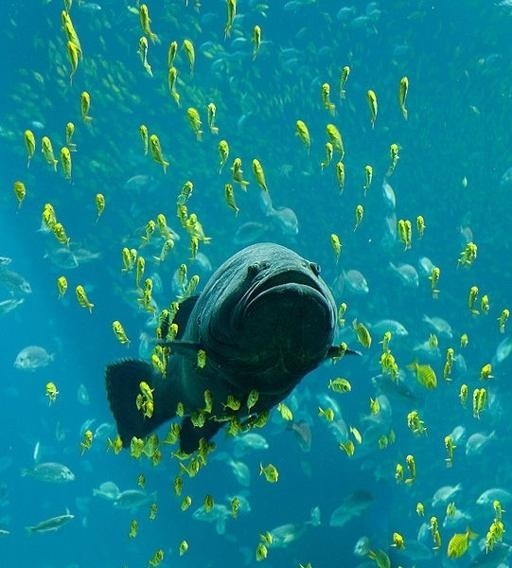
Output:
[0,0,512,567]
